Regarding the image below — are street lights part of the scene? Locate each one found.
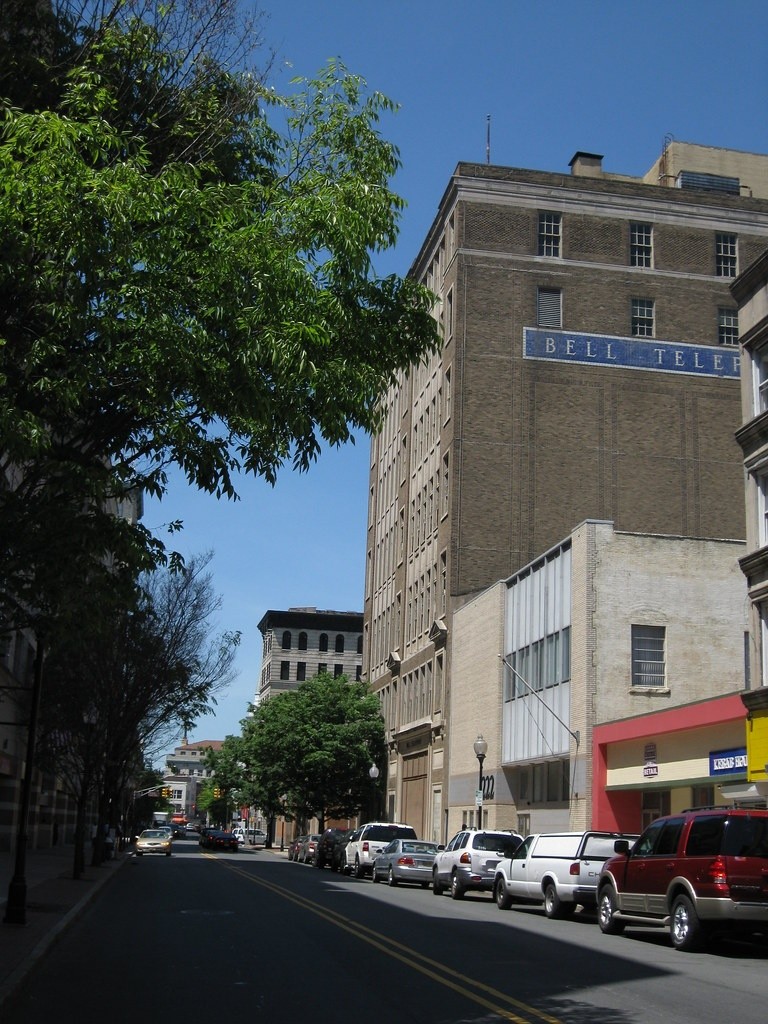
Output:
[473,734,488,830]
[368,763,380,821]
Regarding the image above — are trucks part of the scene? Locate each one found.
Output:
[151,812,170,829]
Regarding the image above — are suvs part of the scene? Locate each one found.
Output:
[597,805,768,948]
[492,830,651,918]
[432,827,529,900]
[315,821,417,878]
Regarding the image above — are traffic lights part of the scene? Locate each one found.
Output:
[192,805,195,809]
[162,789,169,799]
[214,789,221,798]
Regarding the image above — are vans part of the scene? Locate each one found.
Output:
[231,828,267,845]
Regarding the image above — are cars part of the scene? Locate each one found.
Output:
[135,830,173,856]
[372,839,438,888]
[186,822,239,853]
[288,834,322,863]
[159,813,191,839]
